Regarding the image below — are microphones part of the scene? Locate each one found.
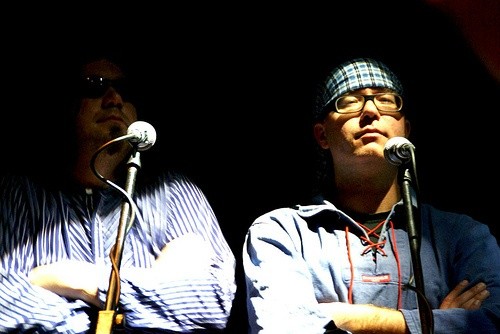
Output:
[384,136,416,167]
[126,120,157,152]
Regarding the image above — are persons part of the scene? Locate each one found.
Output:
[0,49,238,334]
[242,59,500,334]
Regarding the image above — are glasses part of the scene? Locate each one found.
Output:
[329,92,403,113]
[79,74,137,101]
